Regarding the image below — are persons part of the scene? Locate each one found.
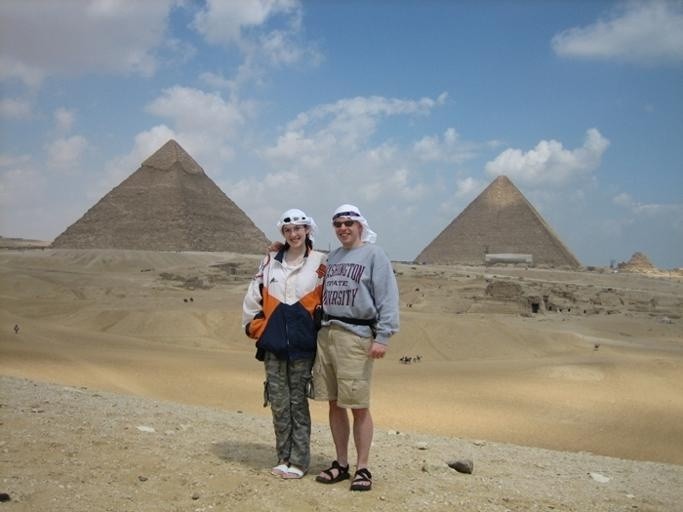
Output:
[241,208,328,479]
[268,205,400,492]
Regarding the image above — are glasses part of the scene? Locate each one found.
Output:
[333,221,353,227]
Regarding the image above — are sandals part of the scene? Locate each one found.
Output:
[351,469,371,490]
[317,461,349,483]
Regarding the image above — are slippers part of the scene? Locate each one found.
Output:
[283,466,308,478]
[271,464,288,475]
